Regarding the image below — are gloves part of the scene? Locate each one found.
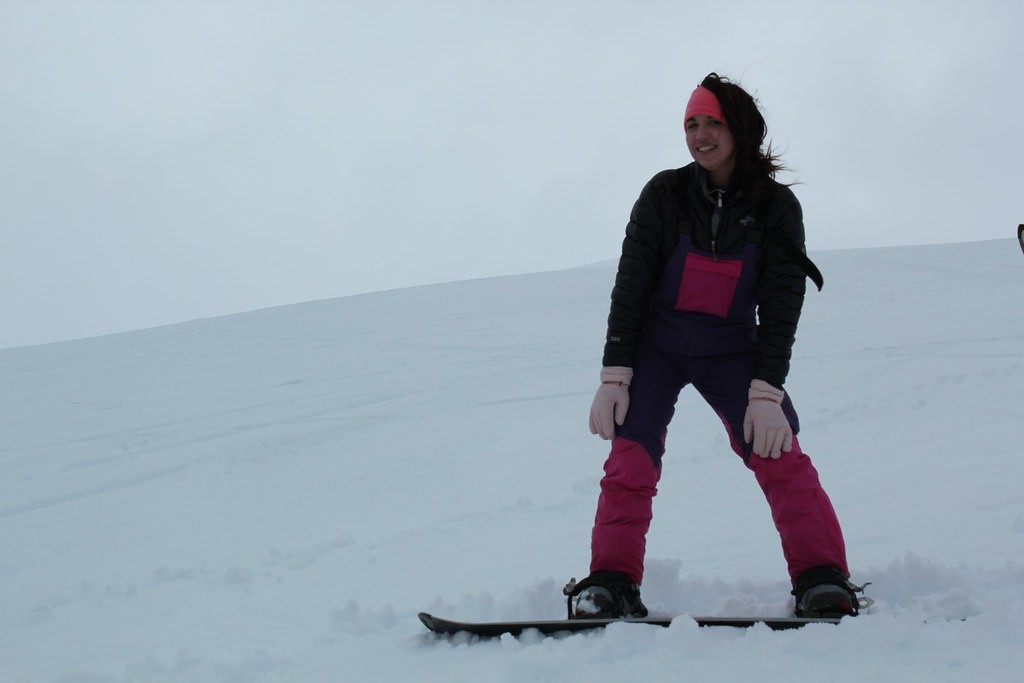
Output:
[590,366,632,440]
[743,379,792,459]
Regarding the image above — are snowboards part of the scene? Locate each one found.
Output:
[419,612,850,637]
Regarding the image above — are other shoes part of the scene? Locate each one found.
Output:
[575,577,640,618]
[799,580,853,617]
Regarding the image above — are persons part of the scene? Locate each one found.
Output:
[573,74,851,620]
